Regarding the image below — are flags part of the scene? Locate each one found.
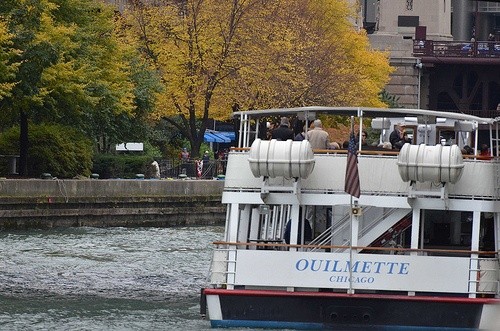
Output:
[344,123,361,198]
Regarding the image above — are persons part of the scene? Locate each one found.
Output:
[350,124,369,150]
[182,147,229,177]
[307,119,329,155]
[271,117,295,142]
[389,120,406,149]
[487,32,496,55]
[283,206,312,251]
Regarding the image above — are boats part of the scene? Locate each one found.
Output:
[200,104,500,331]
